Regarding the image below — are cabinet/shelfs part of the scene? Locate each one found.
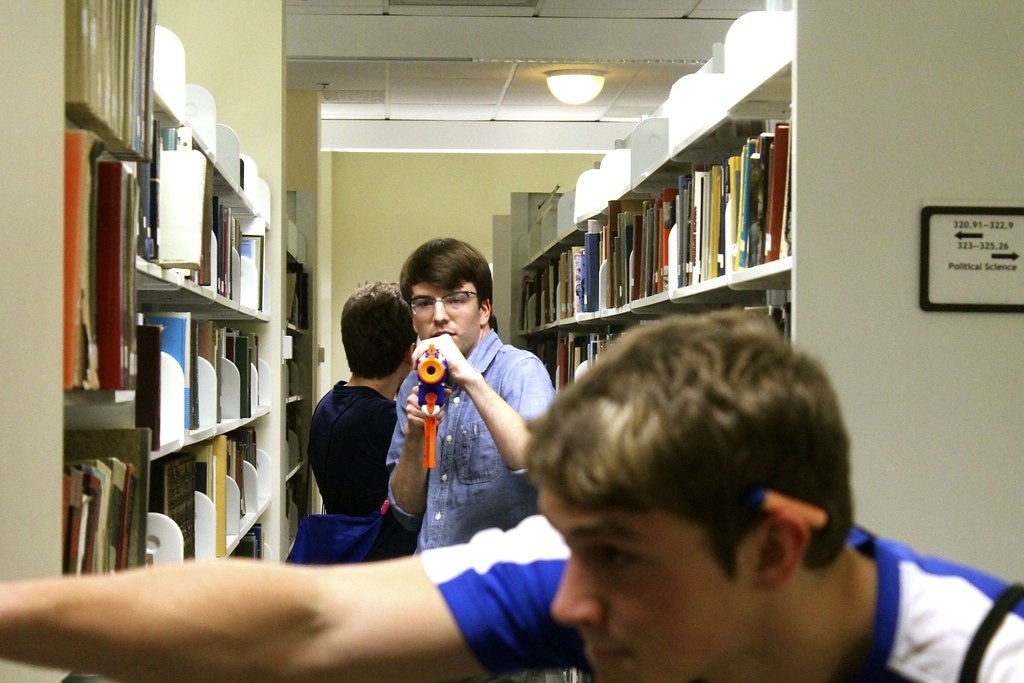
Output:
[1,1,315,683]
[511,61,793,391]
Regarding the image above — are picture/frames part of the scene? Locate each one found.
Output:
[919,205,1024,311]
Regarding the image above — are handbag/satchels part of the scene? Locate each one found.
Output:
[287,511,385,564]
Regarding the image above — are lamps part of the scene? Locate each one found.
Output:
[545,69,605,105]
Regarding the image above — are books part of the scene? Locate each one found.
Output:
[517,240,589,328]
[65,0,162,164]
[137,119,243,298]
[283,260,310,330]
[151,426,266,564]
[62,426,154,576]
[742,300,791,340]
[580,122,793,312]
[133,316,261,451]
[62,129,141,391]
[522,326,627,394]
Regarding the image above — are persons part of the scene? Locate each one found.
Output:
[0,319,1024,683]
[382,239,560,555]
[304,277,418,560]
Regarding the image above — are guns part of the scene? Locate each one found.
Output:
[416,344,448,470]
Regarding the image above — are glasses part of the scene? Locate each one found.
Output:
[410,290,477,317]
